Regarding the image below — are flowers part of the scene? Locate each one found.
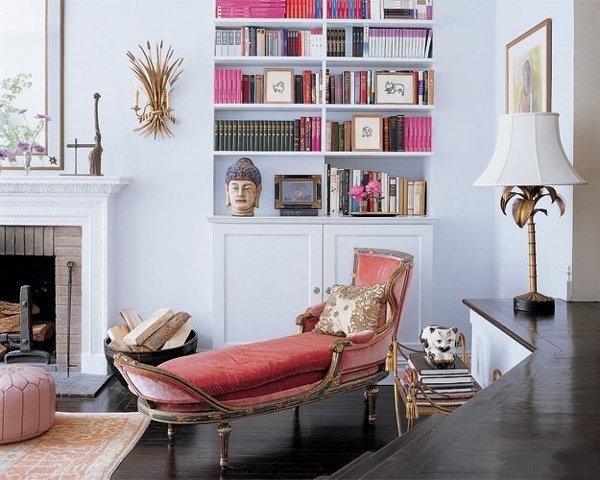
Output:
[347,180,382,203]
[0,104,51,163]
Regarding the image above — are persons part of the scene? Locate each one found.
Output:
[520,62,534,113]
[224,157,262,216]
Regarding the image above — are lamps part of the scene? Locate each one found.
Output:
[472,112,589,318]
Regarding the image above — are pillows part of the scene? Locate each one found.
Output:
[311,282,386,337]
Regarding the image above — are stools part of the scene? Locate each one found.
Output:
[0,364,55,445]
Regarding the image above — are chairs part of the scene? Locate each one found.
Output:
[112,246,415,477]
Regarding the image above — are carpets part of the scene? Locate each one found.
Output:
[1,411,151,480]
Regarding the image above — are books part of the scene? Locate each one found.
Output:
[400,352,475,407]
[326,1,434,58]
[280,208,318,216]
[326,69,434,152]
[215,70,323,151]
[214,1,323,56]
[324,164,426,216]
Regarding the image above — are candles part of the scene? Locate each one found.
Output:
[166,82,170,109]
[135,91,139,106]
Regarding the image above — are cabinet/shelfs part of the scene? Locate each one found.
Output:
[214,1,436,387]
[316,296,599,479]
[393,341,483,437]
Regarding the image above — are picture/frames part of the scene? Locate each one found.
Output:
[275,174,322,216]
[505,18,553,112]
[0,0,64,171]
[374,72,417,105]
[351,114,383,152]
[263,68,295,104]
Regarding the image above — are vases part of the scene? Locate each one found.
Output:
[359,200,368,213]
[24,152,31,174]
